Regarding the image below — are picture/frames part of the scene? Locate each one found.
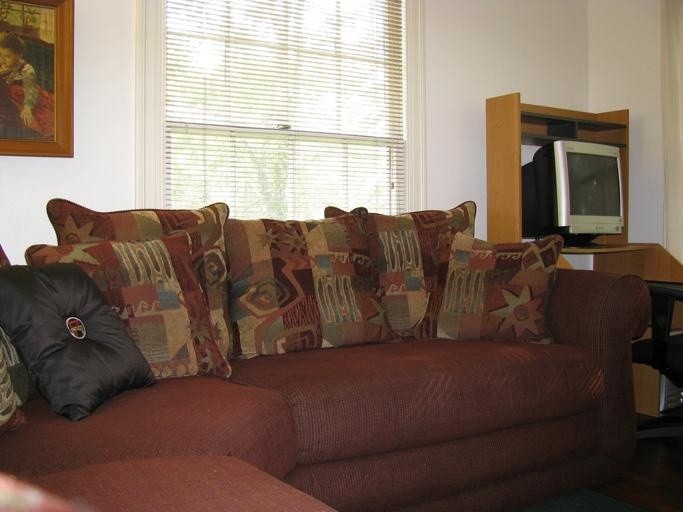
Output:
[0,0,74,158]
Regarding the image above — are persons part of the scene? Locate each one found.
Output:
[0,31,37,128]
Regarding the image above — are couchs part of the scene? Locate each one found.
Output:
[1,245,650,511]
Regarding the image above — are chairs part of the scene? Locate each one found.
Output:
[631,272,682,390]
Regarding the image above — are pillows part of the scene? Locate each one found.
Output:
[224,207,370,357]
[23,232,233,379]
[301,213,436,349]
[44,198,235,361]
[1,262,160,423]
[437,230,561,345]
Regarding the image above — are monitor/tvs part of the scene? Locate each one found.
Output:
[521,139,625,249]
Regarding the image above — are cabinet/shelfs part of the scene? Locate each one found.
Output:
[486,92,682,418]
